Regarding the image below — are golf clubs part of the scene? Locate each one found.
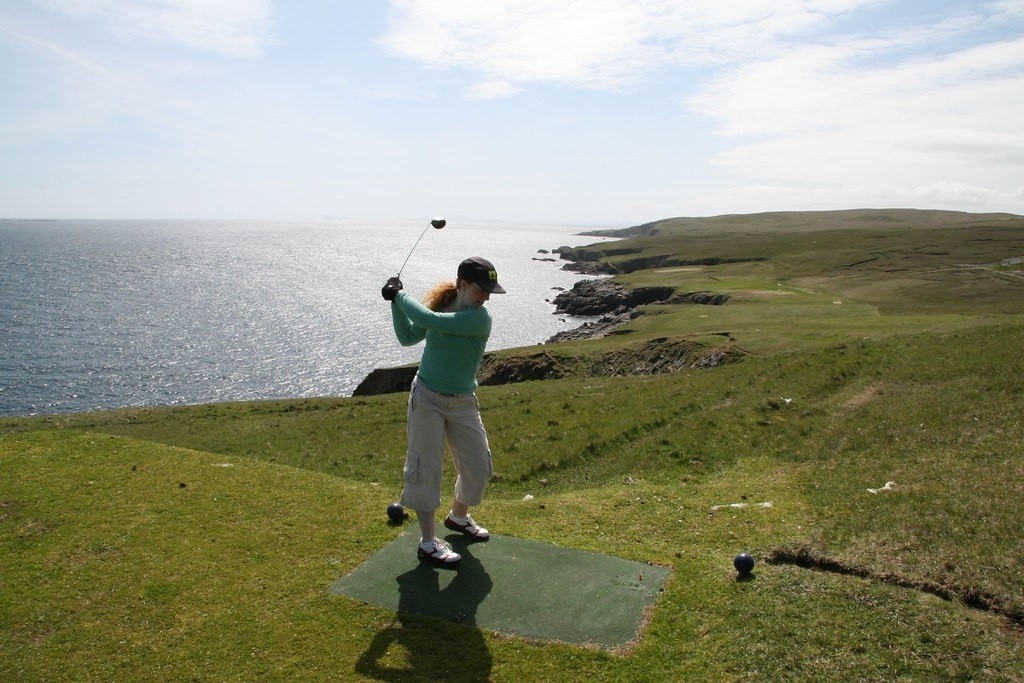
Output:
[395,217,446,282]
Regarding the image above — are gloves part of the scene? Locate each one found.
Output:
[382,277,404,303]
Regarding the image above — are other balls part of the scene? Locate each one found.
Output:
[734,553,755,575]
[387,503,404,522]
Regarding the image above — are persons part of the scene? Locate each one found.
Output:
[382,257,506,564]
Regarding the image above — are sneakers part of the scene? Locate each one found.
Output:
[417,537,462,566]
[444,509,490,539]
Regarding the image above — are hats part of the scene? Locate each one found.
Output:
[458,256,506,294]
